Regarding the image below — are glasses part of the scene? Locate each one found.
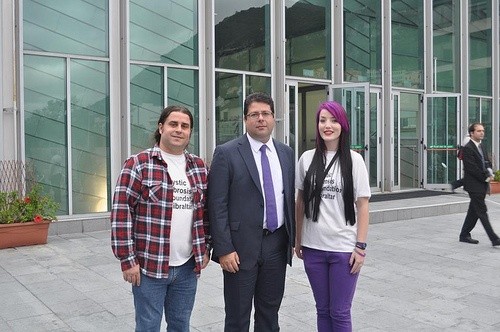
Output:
[245,111,274,119]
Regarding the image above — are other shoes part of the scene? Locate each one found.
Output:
[459,237,479,244]
[492,239,500,246]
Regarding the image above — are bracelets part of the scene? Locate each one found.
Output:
[354,242,367,256]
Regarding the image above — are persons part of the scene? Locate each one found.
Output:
[204,92,296,332]
[294,101,372,332]
[110,105,212,332]
[450,123,500,246]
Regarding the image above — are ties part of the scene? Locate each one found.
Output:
[259,144,279,233]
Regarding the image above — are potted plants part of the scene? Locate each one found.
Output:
[0,179,57,250]
[490,170,500,193]
